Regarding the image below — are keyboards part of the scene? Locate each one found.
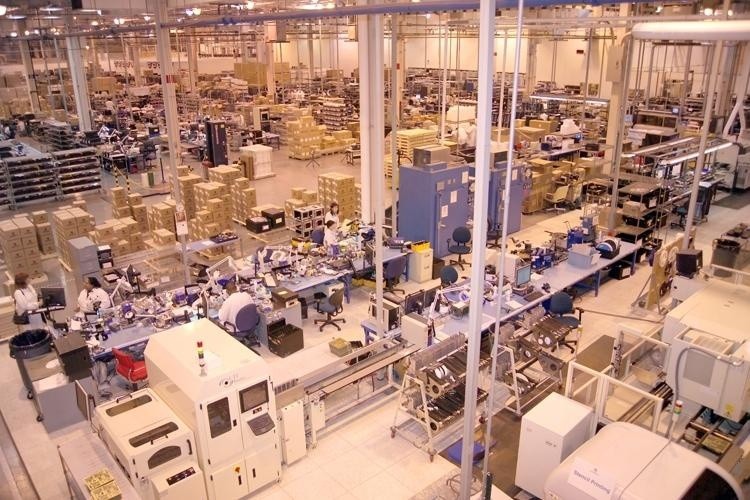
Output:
[524,291,544,302]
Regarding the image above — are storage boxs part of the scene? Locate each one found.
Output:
[0,58,558,178]
[0,164,362,295]
[521,157,590,214]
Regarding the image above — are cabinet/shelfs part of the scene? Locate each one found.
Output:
[582,179,675,269]
[406,248,433,284]
[579,143,614,179]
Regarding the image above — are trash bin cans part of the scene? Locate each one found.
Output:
[9,329,51,400]
[693,181,714,225]
[112,154,126,176]
[709,238,740,276]
[97,245,113,269]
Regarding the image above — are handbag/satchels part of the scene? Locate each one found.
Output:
[12,288,30,325]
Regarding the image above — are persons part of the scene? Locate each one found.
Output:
[219,283,254,332]
[105,96,115,110]
[323,220,337,246]
[13,272,44,334]
[0,118,27,139]
[77,275,111,311]
[325,202,340,226]
[94,89,110,98]
[465,120,476,147]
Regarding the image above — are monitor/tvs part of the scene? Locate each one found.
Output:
[239,380,269,413]
[516,263,531,287]
[201,291,209,319]
[672,107,680,115]
[41,287,66,307]
[126,264,135,283]
[366,241,375,266]
[405,286,441,314]
[75,380,92,422]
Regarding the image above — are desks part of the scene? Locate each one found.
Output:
[413,236,641,345]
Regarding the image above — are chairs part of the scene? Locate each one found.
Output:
[544,184,570,215]
[82,226,413,391]
[446,227,472,271]
[542,292,584,353]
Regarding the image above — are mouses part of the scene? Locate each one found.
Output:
[545,289,550,293]
